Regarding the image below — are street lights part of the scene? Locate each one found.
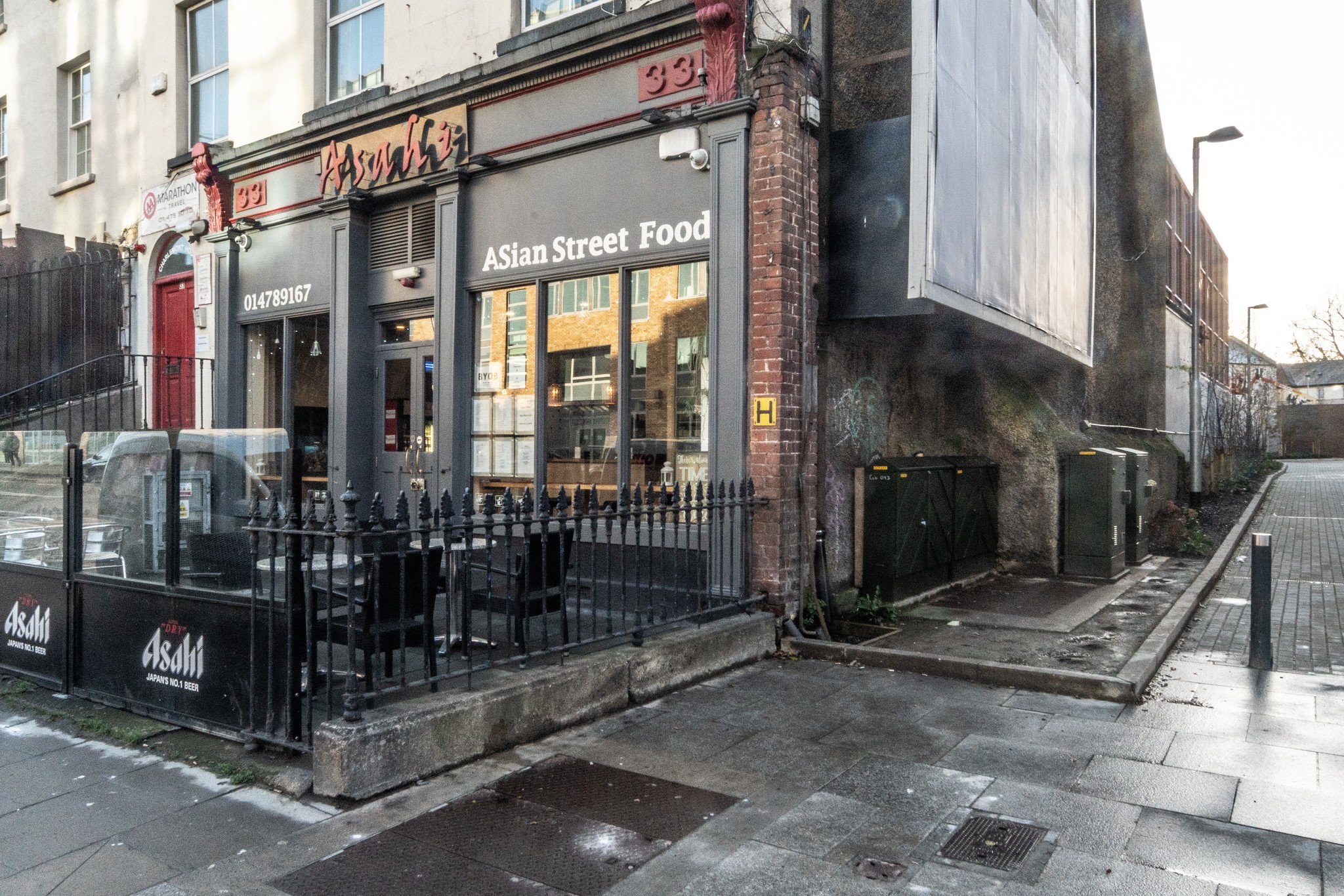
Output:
[1188,125,1243,515]
[1247,304,1269,456]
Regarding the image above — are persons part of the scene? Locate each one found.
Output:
[0,436,11,464]
[7,432,22,467]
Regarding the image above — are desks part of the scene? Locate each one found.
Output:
[257,553,365,696]
[410,537,498,654]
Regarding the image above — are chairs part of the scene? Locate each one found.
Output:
[0,516,132,582]
[182,519,576,710]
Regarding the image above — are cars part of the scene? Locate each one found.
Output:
[82,443,115,483]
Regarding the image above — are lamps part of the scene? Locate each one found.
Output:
[638,108,668,125]
[547,383,564,407]
[347,187,369,199]
[310,318,321,356]
[469,154,496,167]
[235,217,258,226]
[660,461,674,486]
